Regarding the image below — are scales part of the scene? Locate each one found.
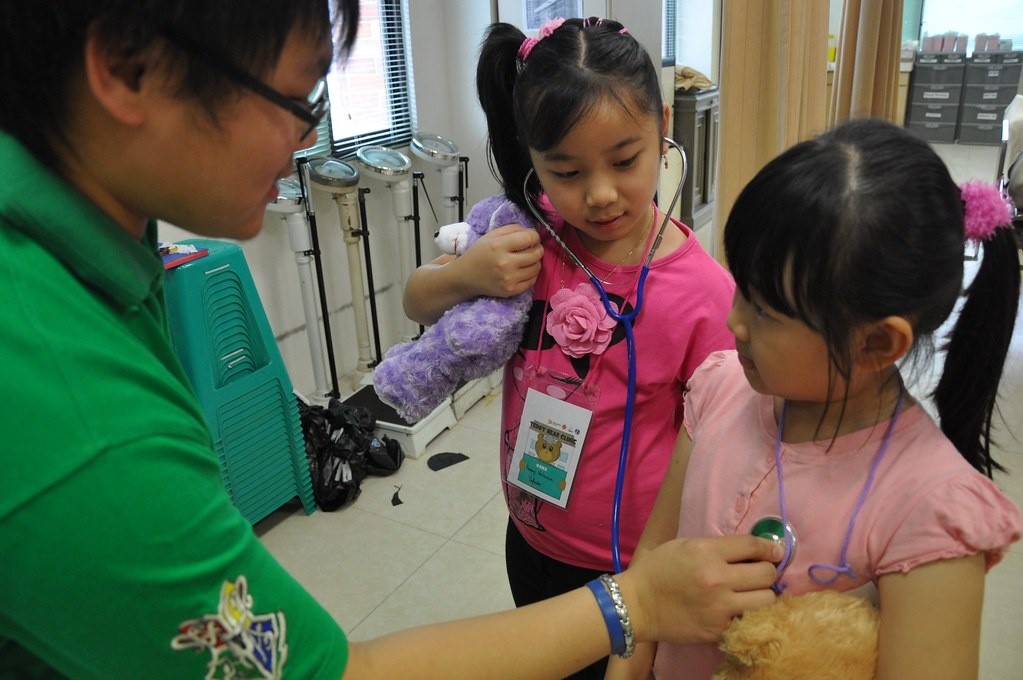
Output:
[265,132,506,461]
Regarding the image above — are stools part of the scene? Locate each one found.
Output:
[164,238,317,527]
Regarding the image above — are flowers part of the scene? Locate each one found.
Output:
[547,282,620,359]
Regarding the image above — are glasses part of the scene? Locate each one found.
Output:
[227,66,330,141]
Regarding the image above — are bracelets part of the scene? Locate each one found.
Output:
[588,573,634,658]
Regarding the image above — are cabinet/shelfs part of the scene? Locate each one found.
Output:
[825,71,909,129]
[674,90,719,231]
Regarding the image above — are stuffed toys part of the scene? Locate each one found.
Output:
[373,193,541,426]
[708,591,882,679]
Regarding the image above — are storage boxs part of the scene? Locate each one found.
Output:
[901,36,1023,146]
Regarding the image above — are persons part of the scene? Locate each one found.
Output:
[0,0,784,680]
[402,17,737,679]
[604,118,1023,679]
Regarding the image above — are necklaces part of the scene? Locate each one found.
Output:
[561,204,653,290]
